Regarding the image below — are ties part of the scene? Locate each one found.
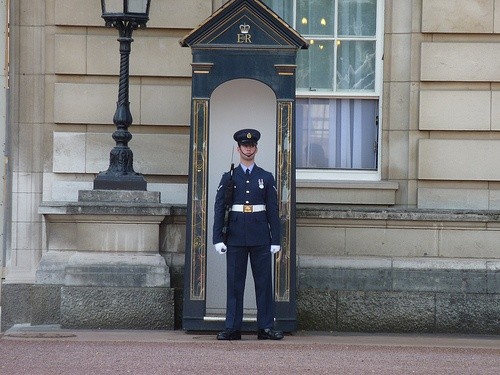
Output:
[245,168,250,181]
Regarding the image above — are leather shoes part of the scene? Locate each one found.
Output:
[258,328,284,340]
[217,328,241,340]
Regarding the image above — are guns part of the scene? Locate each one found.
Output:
[222,144,234,252]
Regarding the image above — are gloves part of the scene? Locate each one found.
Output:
[214,242,227,254]
[271,245,280,254]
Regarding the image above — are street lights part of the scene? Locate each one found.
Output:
[92,0,152,191]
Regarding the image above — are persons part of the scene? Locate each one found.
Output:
[212,129,286,341]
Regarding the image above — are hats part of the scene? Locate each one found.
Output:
[234,129,260,142]
